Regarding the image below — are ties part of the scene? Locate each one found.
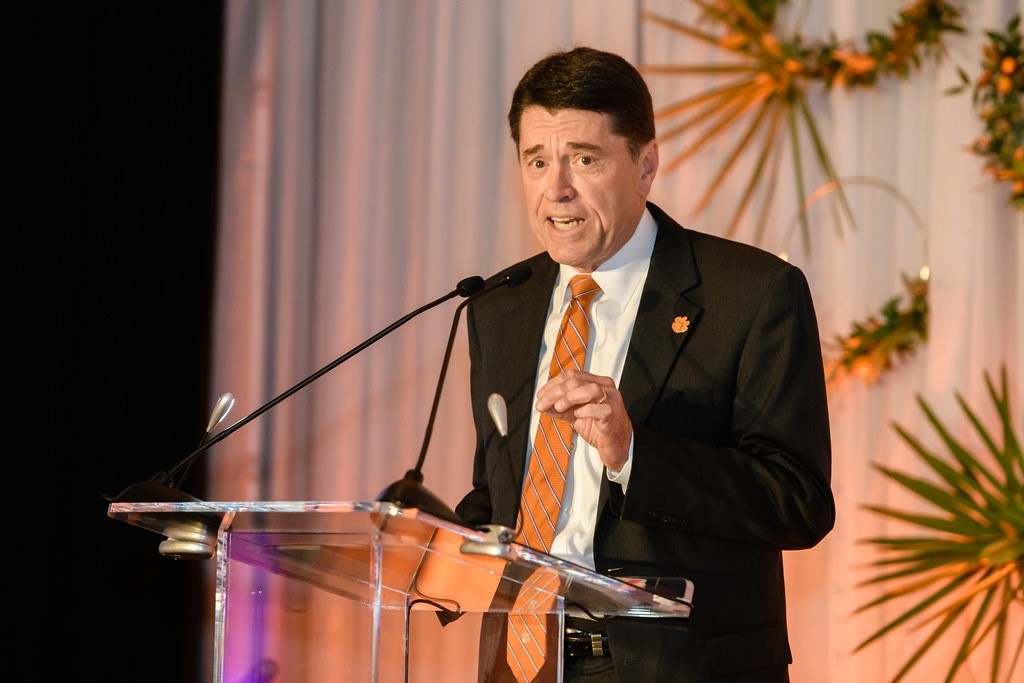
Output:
[507,274,602,683]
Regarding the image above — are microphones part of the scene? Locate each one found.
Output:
[112,276,486,501]
[375,263,533,527]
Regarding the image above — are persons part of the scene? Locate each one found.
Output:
[451,44,837,683]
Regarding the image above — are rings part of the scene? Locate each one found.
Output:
[598,386,606,404]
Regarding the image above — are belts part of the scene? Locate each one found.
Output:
[564,628,612,657]
